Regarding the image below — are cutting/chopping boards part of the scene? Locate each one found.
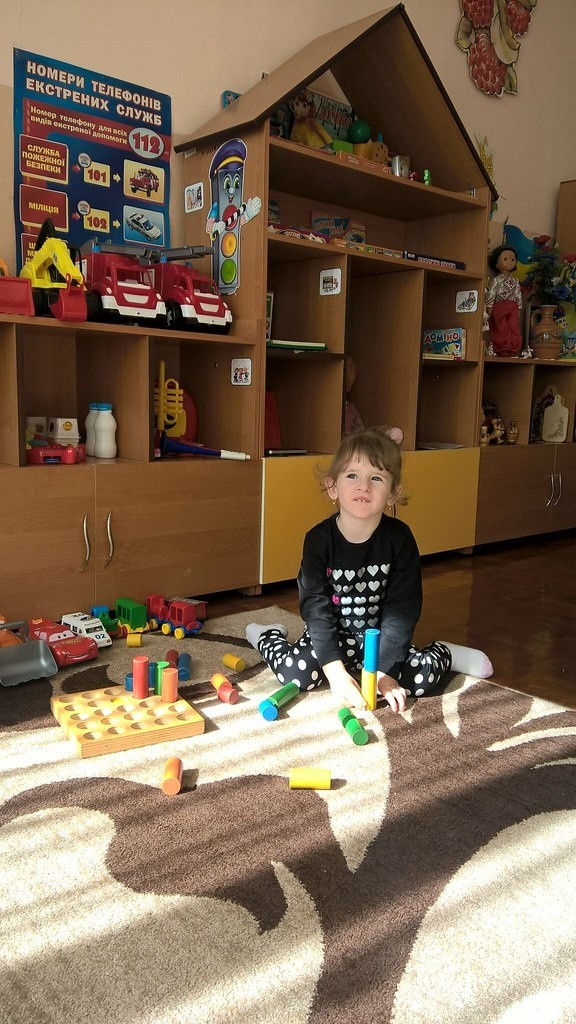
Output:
[542,395,569,444]
[532,385,566,442]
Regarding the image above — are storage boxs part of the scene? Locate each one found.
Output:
[268,338,329,351]
[262,201,469,275]
[334,149,393,175]
[265,289,274,343]
[422,327,467,361]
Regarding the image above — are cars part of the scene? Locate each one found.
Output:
[24,619,99,665]
[126,213,162,241]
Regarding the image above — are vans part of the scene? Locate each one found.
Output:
[61,612,113,648]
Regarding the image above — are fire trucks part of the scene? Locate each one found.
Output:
[129,168,160,197]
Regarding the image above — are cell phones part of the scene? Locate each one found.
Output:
[264,448,308,454]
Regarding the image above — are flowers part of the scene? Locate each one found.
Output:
[517,228,576,308]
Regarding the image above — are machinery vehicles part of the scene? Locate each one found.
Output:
[153,245,232,336]
[0,615,59,687]
[0,258,36,317]
[75,238,166,327]
[20,217,88,323]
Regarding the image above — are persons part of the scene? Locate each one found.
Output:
[246,430,493,697]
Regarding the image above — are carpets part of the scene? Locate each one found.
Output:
[0,602,576,1023]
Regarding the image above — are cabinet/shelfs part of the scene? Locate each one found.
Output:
[173,106,494,597]
[0,312,264,621]
[449,331,576,557]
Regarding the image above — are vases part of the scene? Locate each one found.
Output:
[528,303,563,361]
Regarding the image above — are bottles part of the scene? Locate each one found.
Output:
[94,404,117,458]
[85,404,98,456]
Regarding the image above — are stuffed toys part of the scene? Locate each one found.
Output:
[341,356,404,445]
[488,246,524,359]
